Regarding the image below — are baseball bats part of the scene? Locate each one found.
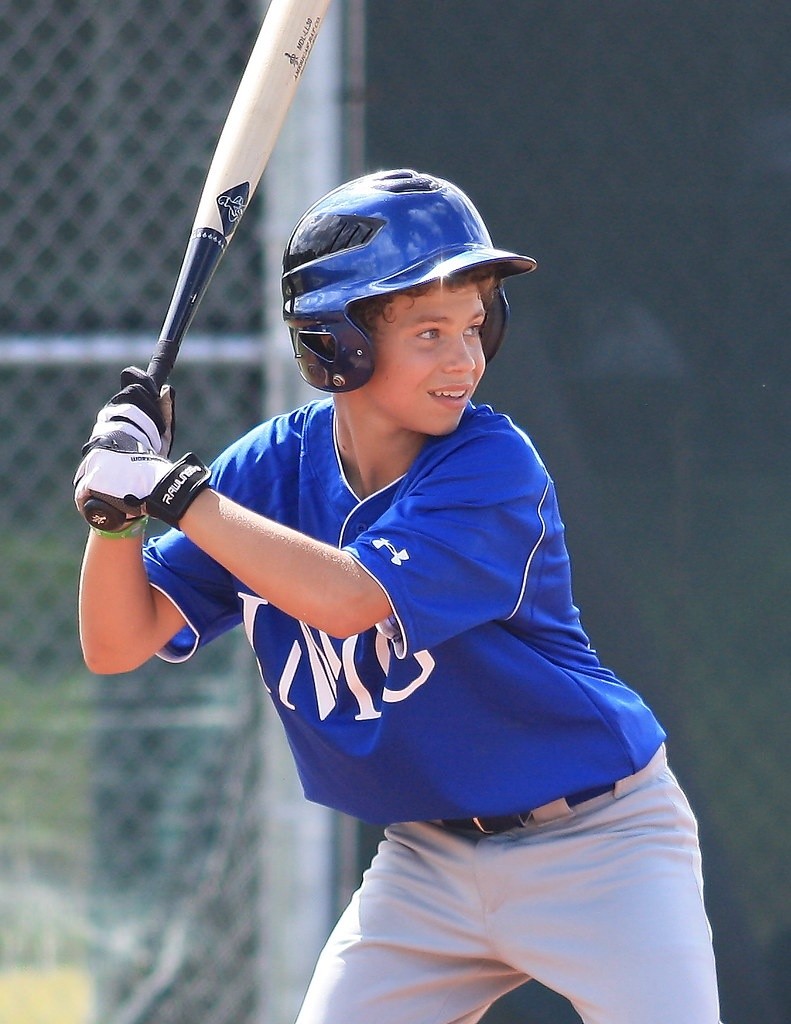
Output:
[80,0,331,533]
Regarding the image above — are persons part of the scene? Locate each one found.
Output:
[74,169,721,1024]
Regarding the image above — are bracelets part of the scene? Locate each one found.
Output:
[90,515,150,540]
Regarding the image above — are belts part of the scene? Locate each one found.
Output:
[441,783,617,834]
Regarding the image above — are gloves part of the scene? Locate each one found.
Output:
[73,365,176,459]
[72,429,212,533]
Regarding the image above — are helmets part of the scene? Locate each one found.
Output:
[281,170,536,393]
